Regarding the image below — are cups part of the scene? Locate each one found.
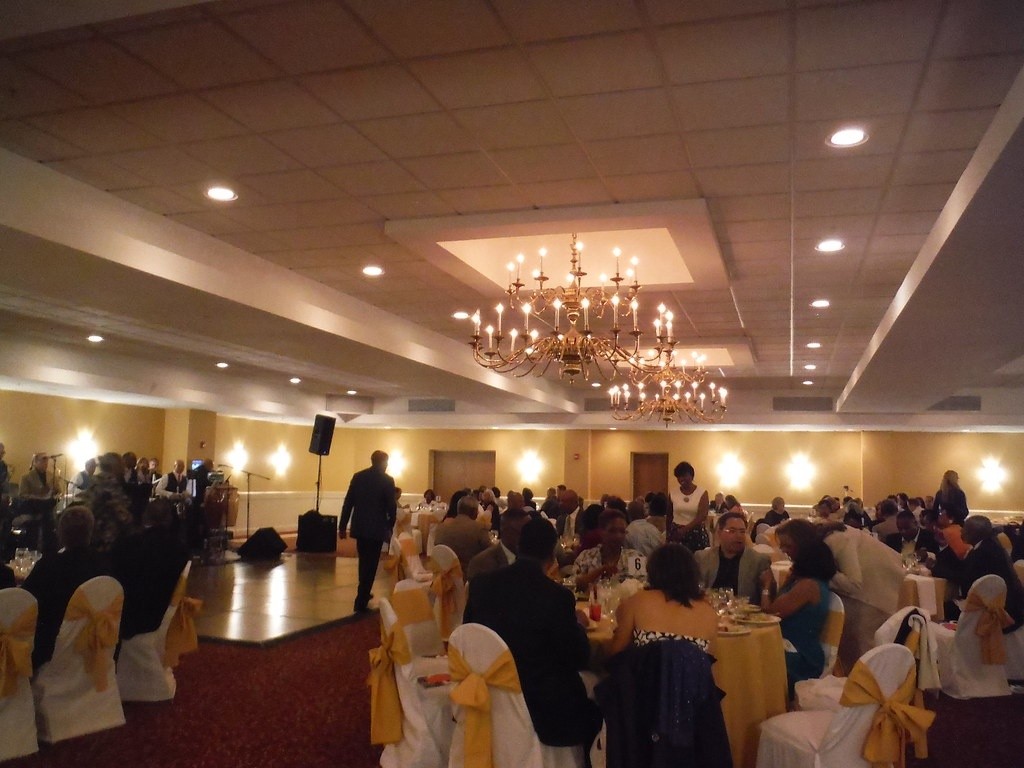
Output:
[553,578,751,626]
[560,533,580,551]
[10,548,43,578]
[900,553,921,572]
[486,530,498,545]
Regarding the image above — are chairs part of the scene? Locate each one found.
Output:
[368,507,1024,768]
[32,575,128,745]
[115,559,203,702]
[1,587,41,760]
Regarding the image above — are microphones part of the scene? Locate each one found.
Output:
[50,453,64,458]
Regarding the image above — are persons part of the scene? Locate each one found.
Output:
[765,497,790,527]
[339,450,397,612]
[395,487,436,509]
[932,470,970,526]
[433,483,603,747]
[82,471,127,518]
[19,505,114,672]
[0,443,214,541]
[92,498,172,663]
[141,493,194,565]
[758,519,836,705]
[872,493,1024,634]
[809,495,876,533]
[573,460,777,768]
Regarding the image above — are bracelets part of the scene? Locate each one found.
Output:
[761,590,771,595]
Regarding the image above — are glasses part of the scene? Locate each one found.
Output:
[724,529,745,535]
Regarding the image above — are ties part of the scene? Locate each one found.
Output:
[178,474,181,480]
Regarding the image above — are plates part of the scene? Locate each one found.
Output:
[718,628,751,634]
[738,615,782,624]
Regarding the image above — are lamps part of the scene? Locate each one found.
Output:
[607,348,728,429]
[470,238,679,384]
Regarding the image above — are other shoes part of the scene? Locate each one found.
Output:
[355,605,368,612]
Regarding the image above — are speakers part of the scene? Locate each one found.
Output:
[309,415,336,455]
[237,527,288,559]
[297,515,337,553]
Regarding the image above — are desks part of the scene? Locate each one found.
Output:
[770,559,959,623]
[573,600,791,768]
